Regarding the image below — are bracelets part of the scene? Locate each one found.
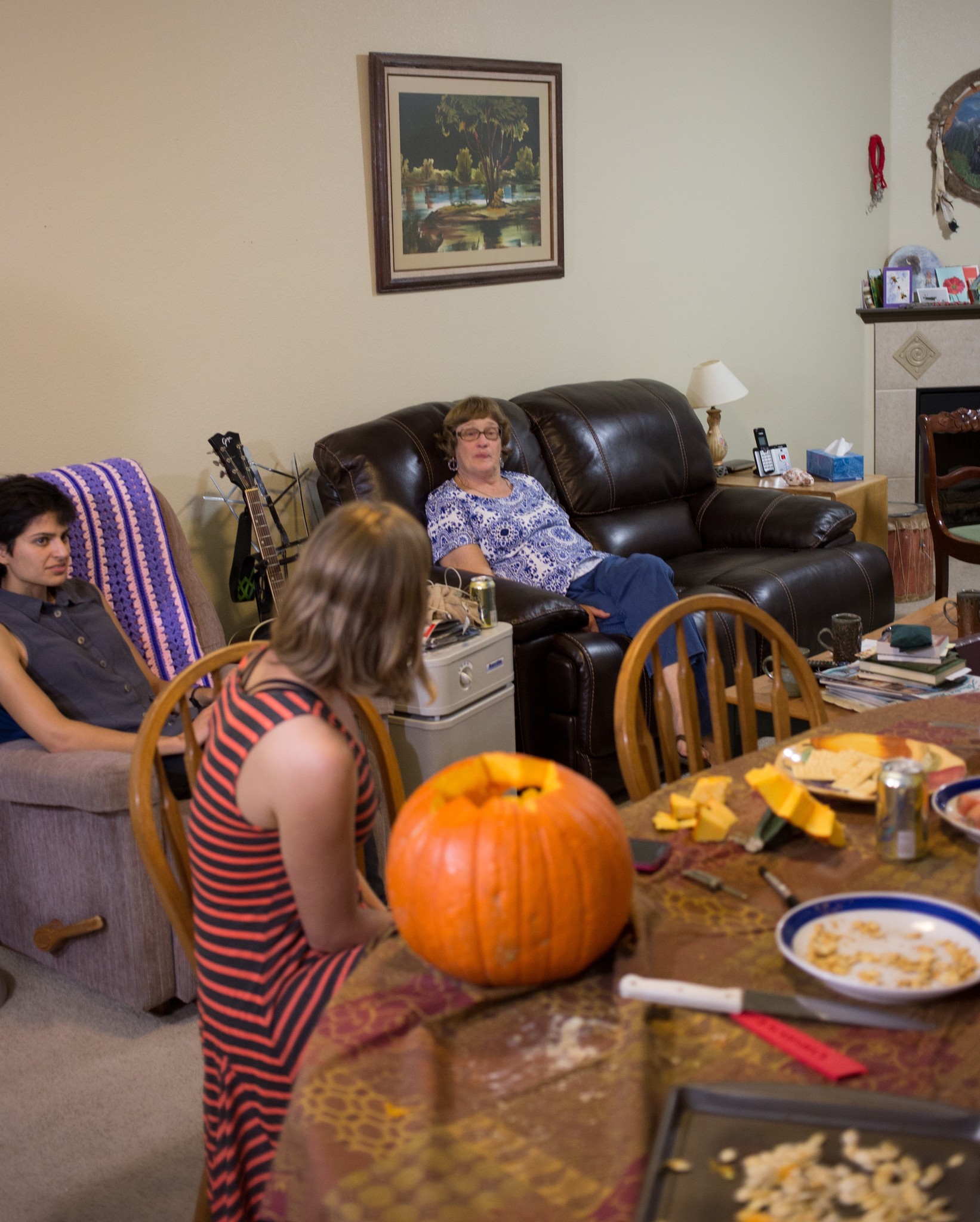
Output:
[189,687,207,707]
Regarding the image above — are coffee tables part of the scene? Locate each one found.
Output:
[721,596,957,760]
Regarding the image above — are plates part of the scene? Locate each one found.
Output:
[774,732,967,802]
[931,775,980,844]
[774,891,980,1004]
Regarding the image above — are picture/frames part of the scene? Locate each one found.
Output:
[928,65,980,207]
[366,51,564,295]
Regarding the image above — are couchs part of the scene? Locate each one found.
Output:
[1,455,240,1020]
[311,379,894,805]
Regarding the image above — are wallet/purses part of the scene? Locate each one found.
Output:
[889,623,933,652]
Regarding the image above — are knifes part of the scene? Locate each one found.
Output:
[619,974,935,1031]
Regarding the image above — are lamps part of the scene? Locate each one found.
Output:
[687,357,751,469]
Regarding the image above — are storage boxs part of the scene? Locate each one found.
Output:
[806,448,864,481]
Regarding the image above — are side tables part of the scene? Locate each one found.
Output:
[713,465,888,556]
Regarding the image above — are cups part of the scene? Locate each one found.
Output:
[762,647,810,697]
[817,612,862,662]
[944,589,980,639]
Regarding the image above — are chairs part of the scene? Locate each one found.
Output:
[612,594,829,804]
[127,639,406,986]
[914,406,980,603]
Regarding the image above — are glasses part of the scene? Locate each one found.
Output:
[455,426,502,441]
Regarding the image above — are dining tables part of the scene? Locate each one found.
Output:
[262,689,977,1220]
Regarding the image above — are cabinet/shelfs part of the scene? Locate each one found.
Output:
[370,620,517,792]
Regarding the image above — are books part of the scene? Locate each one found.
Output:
[813,631,979,713]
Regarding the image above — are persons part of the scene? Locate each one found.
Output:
[191,495,439,1222]
[0,475,214,801]
[423,396,718,766]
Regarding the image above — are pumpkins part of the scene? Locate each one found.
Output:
[374,752,642,996]
